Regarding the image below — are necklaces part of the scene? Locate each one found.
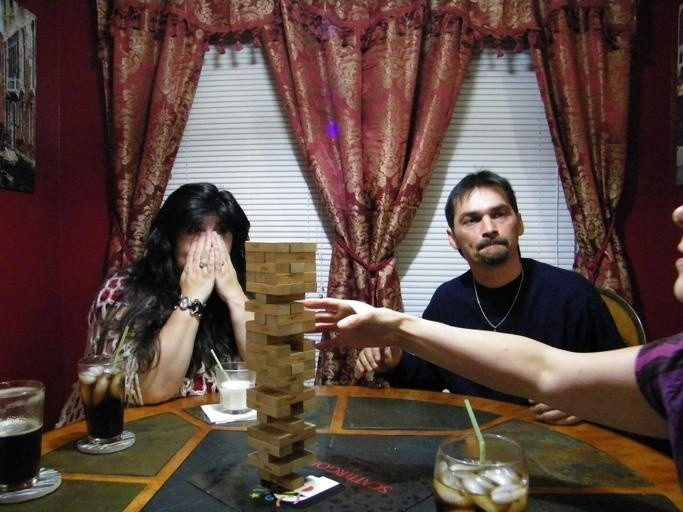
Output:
[472,269,524,332]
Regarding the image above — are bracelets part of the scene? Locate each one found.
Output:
[173,297,207,318]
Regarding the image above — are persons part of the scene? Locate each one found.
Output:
[354,169,627,425]
[55,183,254,428]
[304,193,683,495]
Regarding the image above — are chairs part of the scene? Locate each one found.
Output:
[593,287,649,349]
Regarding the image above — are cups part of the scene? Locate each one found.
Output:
[75,353,125,443]
[431,434,529,511]
[0,379,45,492]
[215,361,256,414]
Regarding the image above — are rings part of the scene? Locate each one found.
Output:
[220,262,224,267]
[198,263,208,268]
